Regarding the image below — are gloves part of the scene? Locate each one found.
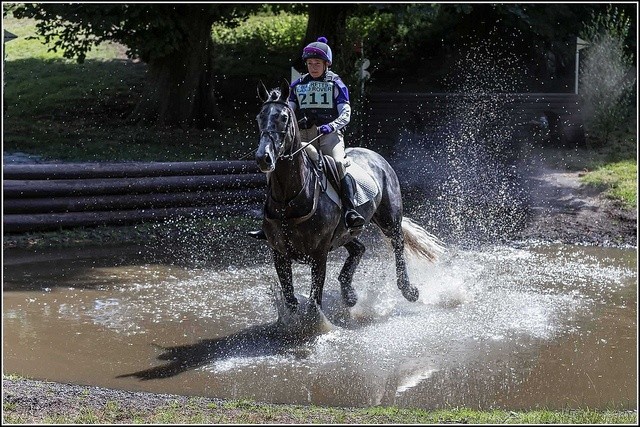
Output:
[316,123,334,137]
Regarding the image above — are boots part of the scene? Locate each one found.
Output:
[248,228,266,239]
[339,172,365,236]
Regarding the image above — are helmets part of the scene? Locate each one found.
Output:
[302,37,332,65]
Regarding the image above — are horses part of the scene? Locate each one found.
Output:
[253,76,447,343]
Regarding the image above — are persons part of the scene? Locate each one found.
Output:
[247,37,366,240]
